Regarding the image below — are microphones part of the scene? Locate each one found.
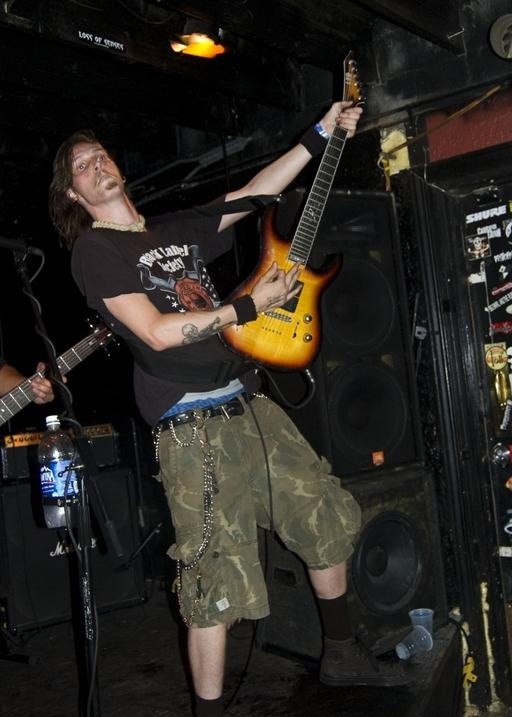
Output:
[0,234,40,255]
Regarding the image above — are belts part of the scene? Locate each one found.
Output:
[155,388,257,433]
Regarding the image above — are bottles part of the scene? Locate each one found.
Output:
[36,414,81,532]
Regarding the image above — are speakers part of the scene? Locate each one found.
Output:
[254,185,428,483]
[255,469,449,661]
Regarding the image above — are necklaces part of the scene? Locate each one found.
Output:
[91,213,149,233]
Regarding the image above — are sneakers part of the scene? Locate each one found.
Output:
[320,636,417,687]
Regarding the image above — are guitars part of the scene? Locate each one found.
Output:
[1,316,121,424]
[215,49,367,367]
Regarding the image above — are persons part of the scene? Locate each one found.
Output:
[1,358,70,420]
[48,97,420,714]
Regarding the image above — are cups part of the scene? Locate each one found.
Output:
[395,623,434,661]
[408,607,435,637]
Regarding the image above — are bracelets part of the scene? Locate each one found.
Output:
[230,292,260,326]
[298,127,330,159]
